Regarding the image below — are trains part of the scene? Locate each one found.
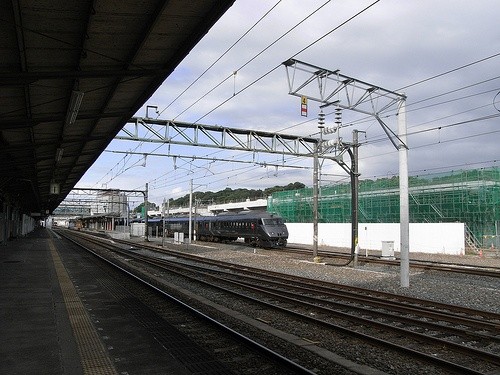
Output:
[69,212,288,250]
